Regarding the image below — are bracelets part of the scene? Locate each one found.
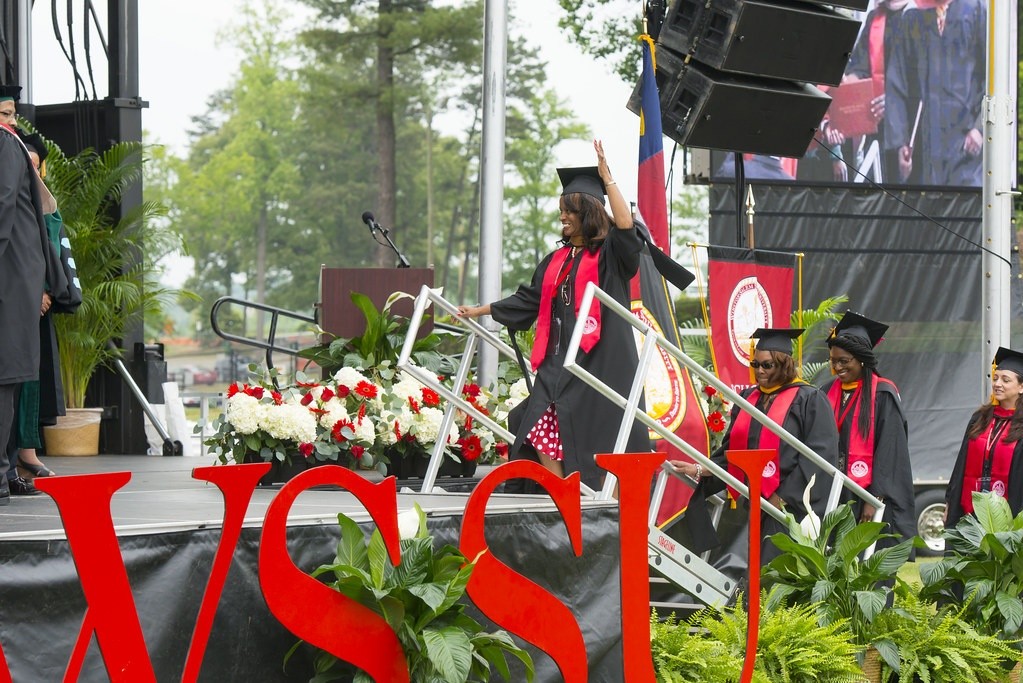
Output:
[779,498,788,506]
[693,463,702,481]
[605,181,616,186]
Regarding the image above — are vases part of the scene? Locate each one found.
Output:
[383,446,479,480]
[245,449,352,487]
[861,648,881,682]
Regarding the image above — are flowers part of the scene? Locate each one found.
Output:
[201,365,536,469]
[759,473,926,670]
[691,372,734,456]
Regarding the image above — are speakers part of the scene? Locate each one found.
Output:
[625,0,869,159]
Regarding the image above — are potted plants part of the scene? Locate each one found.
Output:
[14,114,204,456]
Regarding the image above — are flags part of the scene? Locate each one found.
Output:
[638,32,671,256]
[707,244,795,409]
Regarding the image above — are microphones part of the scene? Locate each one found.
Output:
[362,211,376,237]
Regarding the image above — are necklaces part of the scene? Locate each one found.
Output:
[763,395,769,405]
[571,247,576,258]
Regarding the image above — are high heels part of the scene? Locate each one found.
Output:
[16,455,55,480]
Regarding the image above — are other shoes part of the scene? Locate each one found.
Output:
[11,476,43,495]
[0,487,11,497]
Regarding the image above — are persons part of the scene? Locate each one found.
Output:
[666,328,838,575]
[945,346,1023,604]
[714,0,986,187]
[449,139,696,497]
[0,85,83,507]
[818,311,918,562]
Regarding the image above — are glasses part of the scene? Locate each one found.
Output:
[562,275,571,306]
[827,357,854,365]
[750,359,775,369]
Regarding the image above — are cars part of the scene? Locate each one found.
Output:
[166,364,218,389]
[214,355,261,380]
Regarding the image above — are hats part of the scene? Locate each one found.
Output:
[748,328,806,384]
[992,347,1023,405]
[15,129,48,169]
[0,85,21,102]
[557,165,612,206]
[825,311,890,376]
